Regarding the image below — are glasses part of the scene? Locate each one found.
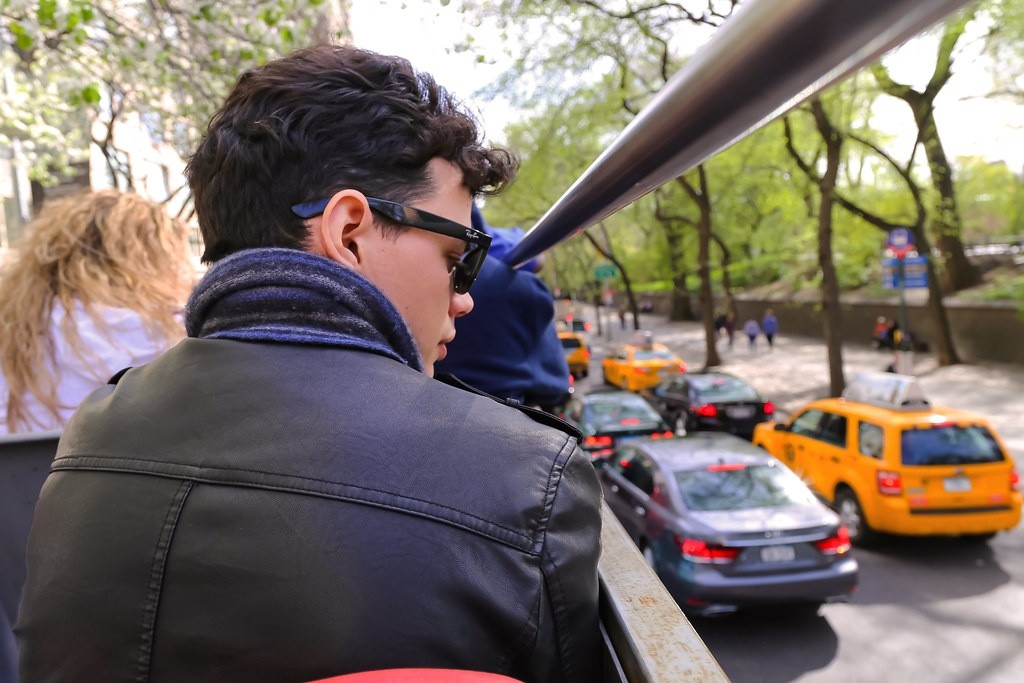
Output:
[292,197,493,294]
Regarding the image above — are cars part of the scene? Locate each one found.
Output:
[566,390,675,469]
[596,431,859,623]
[602,331,686,392]
[554,313,594,381]
[653,372,774,442]
[752,373,1022,544]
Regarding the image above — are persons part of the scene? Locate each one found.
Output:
[0,44,604,683]
[712,307,778,351]
[619,299,653,328]
[870,313,919,353]
